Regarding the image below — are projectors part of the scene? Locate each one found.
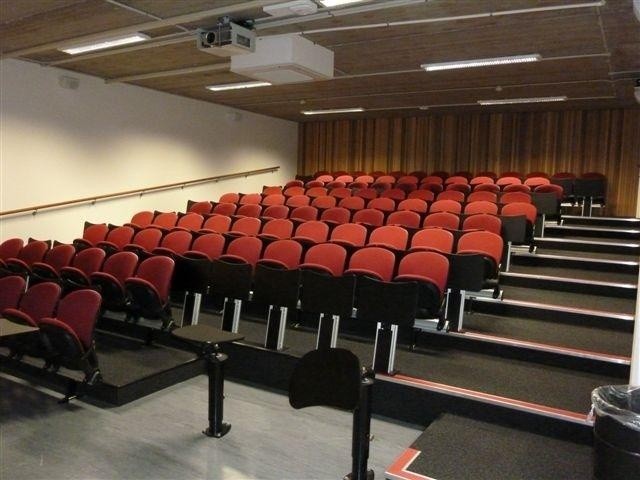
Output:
[195,24,256,57]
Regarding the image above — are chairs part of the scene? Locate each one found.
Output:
[0,238,174,403]
[82,171,605,377]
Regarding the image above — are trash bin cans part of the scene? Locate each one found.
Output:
[591,385,640,480]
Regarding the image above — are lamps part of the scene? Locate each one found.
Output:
[418,53,543,72]
[56,31,152,56]
[206,79,274,92]
